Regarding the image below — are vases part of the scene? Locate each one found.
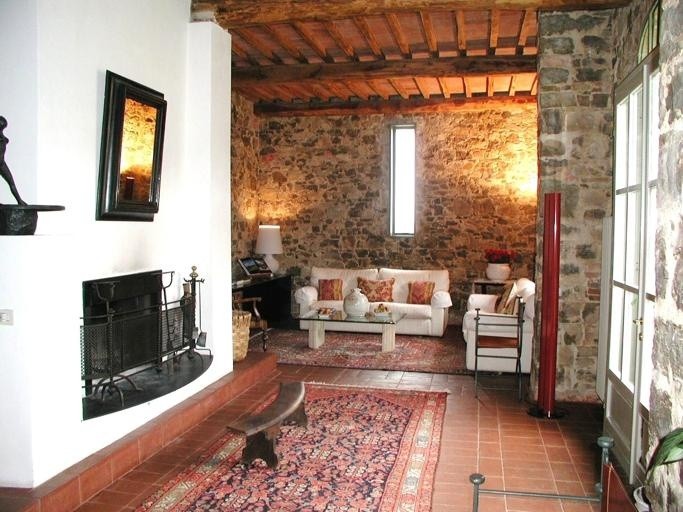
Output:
[486,263,511,283]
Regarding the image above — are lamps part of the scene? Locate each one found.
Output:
[255,225,284,277]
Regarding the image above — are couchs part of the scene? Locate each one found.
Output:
[462,278,535,375]
[294,266,452,337]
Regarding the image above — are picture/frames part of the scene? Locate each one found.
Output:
[92,70,166,222]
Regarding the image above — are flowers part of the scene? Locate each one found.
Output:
[485,247,517,264]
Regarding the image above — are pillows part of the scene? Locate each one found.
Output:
[406,281,435,304]
[357,277,395,302]
[318,279,344,300]
[494,283,515,313]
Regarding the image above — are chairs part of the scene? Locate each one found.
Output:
[232,292,267,353]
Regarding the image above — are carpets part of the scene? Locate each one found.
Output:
[248,329,493,375]
[129,381,447,512]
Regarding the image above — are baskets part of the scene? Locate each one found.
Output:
[232,309,252,363]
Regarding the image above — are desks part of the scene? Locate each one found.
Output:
[232,274,292,328]
